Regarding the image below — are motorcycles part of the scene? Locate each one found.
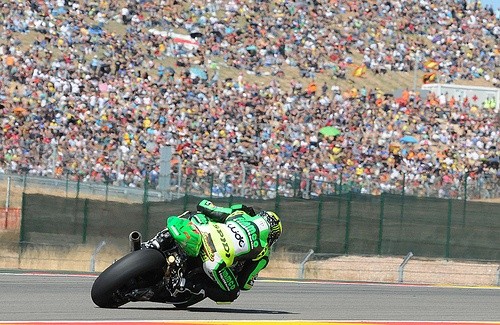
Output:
[89,201,260,311]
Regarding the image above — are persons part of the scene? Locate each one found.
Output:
[0,0,500,201]
[141,199,283,310]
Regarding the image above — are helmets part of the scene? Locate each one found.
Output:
[260,210,282,242]
